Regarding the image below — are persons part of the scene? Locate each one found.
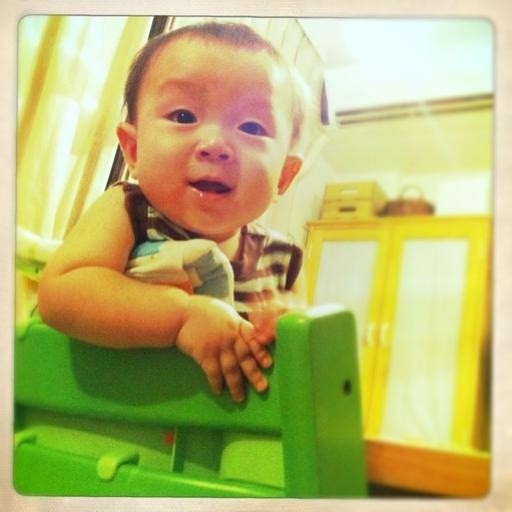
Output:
[37,22,305,402]
